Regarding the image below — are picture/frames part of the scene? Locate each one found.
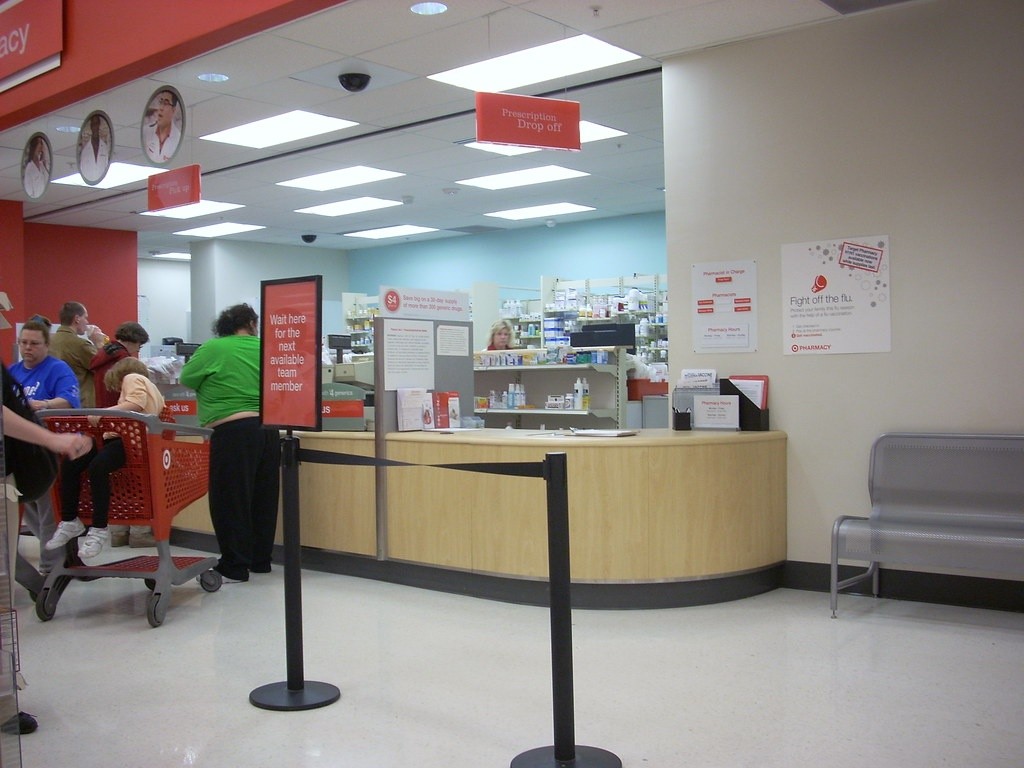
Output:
[140,84,187,168]
[20,132,54,202]
[75,109,114,186]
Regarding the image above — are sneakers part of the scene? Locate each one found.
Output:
[45,516,86,551]
[77,524,111,558]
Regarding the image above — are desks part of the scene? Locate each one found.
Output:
[174,423,794,614]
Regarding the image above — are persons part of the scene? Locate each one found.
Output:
[1,353,92,735]
[23,139,49,198]
[46,355,166,558]
[185,303,280,580]
[482,319,516,352]
[141,91,180,162]
[9,303,165,551]
[79,116,111,183]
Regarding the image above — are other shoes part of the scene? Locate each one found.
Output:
[1,711,39,734]
[196,568,245,584]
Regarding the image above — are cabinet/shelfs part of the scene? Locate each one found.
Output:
[347,307,378,355]
[472,346,624,430]
[499,301,542,350]
[544,279,665,366]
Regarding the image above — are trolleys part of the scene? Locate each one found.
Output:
[34,407,223,628]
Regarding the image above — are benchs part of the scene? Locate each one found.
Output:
[829,430,1024,619]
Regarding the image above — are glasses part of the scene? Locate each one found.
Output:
[18,339,44,347]
[159,100,174,107]
